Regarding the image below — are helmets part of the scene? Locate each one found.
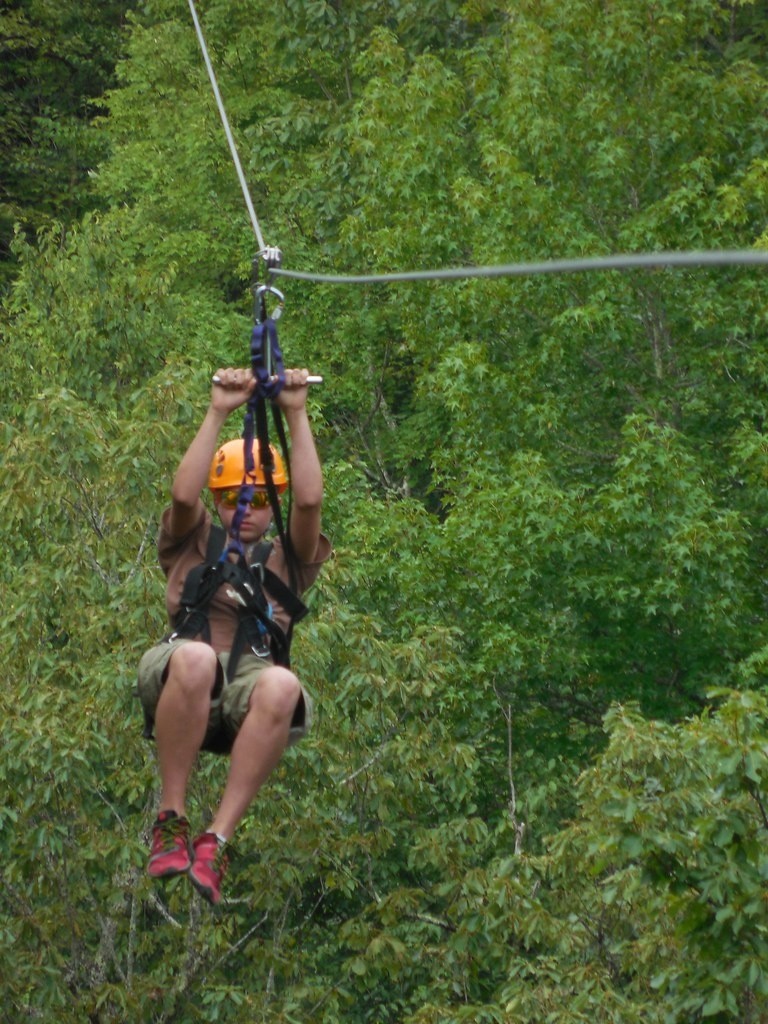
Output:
[207,439,287,494]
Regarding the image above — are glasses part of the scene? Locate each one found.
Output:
[215,489,277,510]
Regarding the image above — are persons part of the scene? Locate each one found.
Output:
[134,368,332,905]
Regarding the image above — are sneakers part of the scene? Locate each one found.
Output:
[188,832,234,905]
[147,810,193,878]
